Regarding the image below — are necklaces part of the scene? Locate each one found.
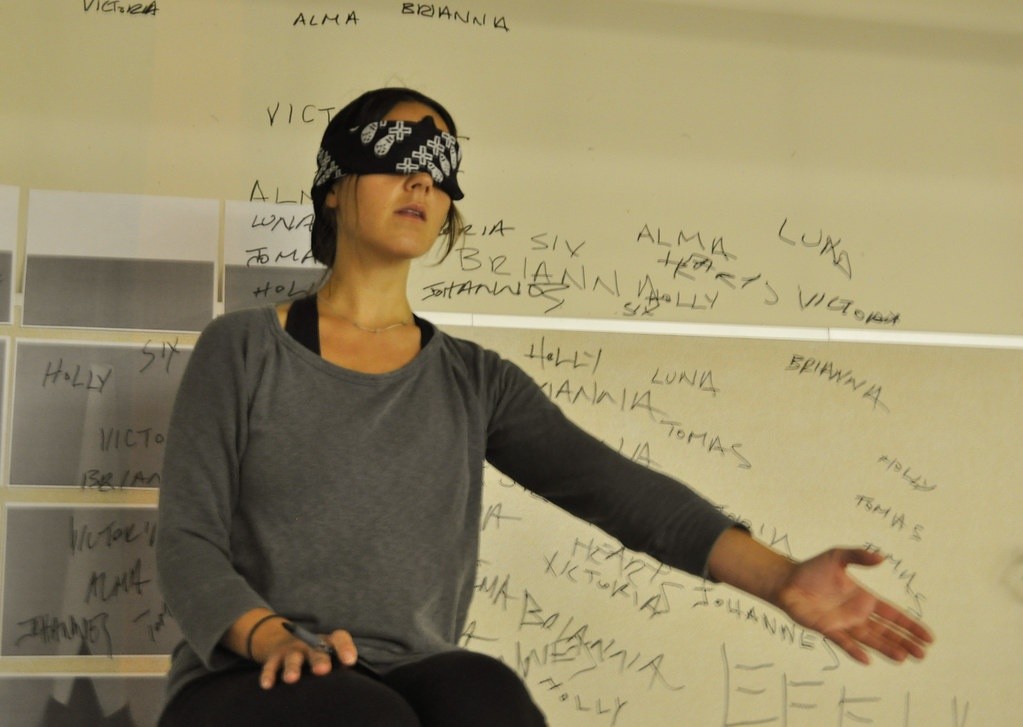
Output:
[315,286,416,332]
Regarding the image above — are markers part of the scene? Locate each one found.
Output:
[283,622,382,681]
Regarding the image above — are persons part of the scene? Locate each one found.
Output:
[148,87,935,727]
